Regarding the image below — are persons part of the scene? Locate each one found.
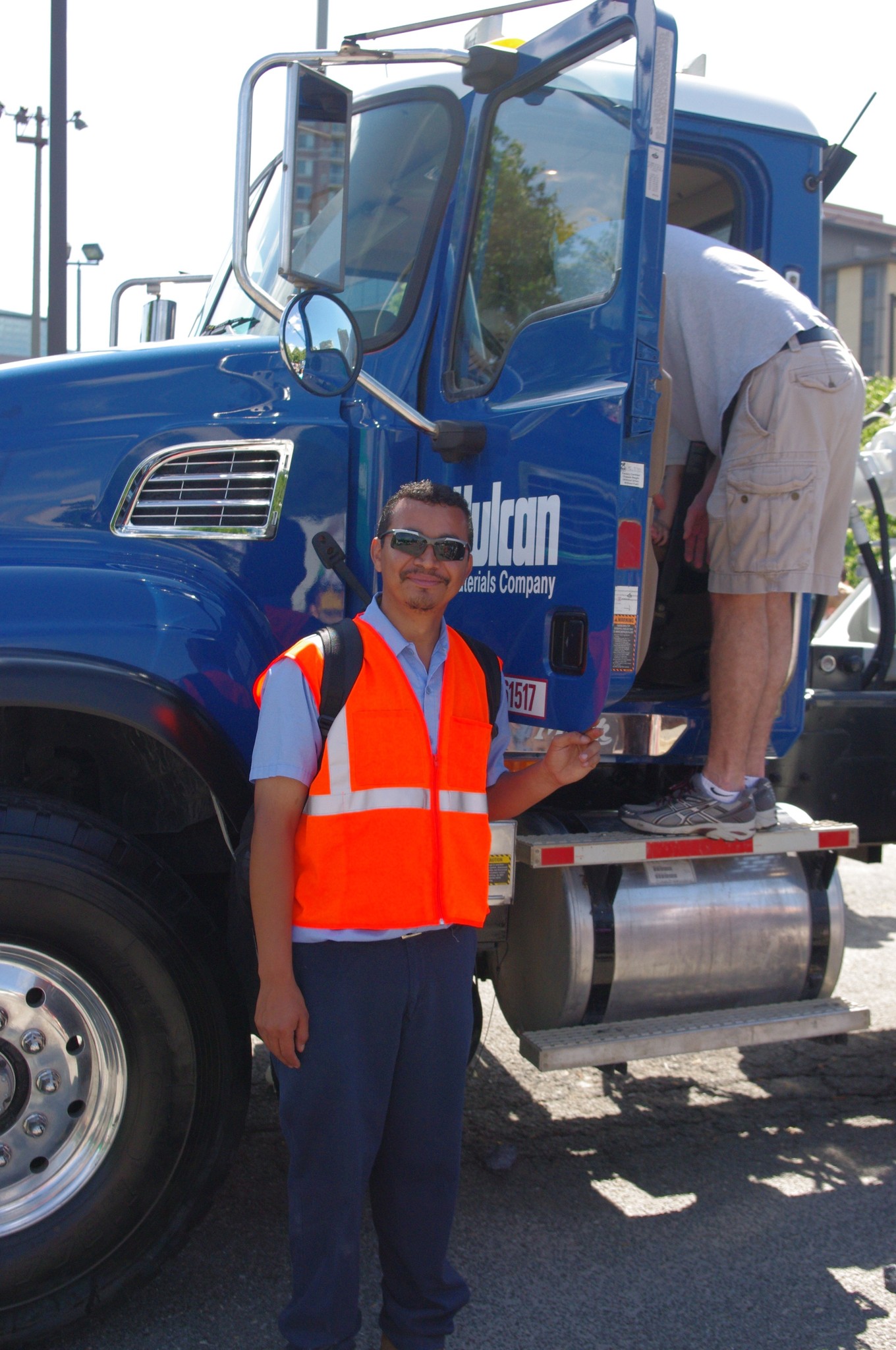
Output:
[252,480,606,1350]
[526,216,865,840]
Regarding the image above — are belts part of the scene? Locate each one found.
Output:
[720,327,842,454]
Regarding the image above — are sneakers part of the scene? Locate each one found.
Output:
[616,775,757,842]
[753,776,778,830]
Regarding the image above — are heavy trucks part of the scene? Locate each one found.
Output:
[0,0,896,1345]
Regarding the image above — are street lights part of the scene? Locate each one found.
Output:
[3,101,102,359]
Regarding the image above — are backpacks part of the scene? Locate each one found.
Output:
[227,617,502,1038]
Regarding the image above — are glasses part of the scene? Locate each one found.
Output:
[378,528,472,563]
